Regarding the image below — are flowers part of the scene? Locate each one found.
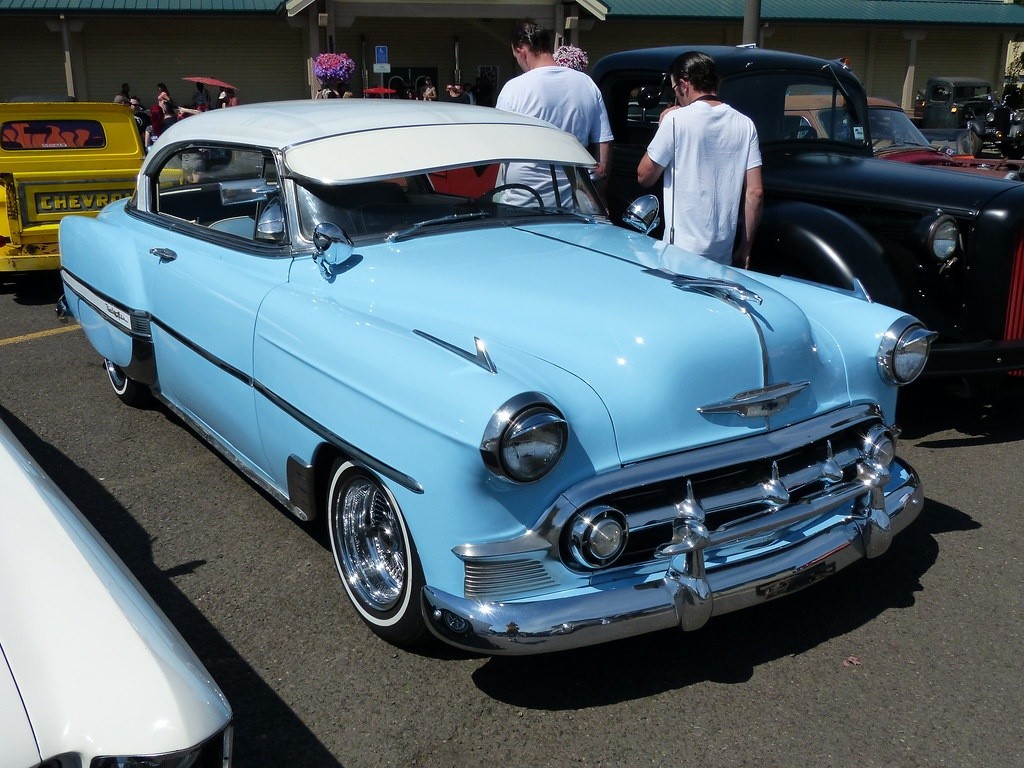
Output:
[313,53,356,83]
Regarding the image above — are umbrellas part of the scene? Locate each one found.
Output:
[181,76,238,90]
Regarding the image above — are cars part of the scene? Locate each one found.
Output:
[54,97,939,658]
[586,46,1024,378]
[783,93,1023,182]
[0,420,237,767]
[907,77,1024,159]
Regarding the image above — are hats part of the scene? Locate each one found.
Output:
[218,91,226,100]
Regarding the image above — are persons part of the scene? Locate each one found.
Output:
[406,77,485,105]
[636,50,763,270]
[494,23,613,211]
[315,84,352,99]
[113,84,238,154]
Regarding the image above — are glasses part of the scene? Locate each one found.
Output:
[671,84,678,91]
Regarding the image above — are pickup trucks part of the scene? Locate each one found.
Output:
[0,97,184,272]
